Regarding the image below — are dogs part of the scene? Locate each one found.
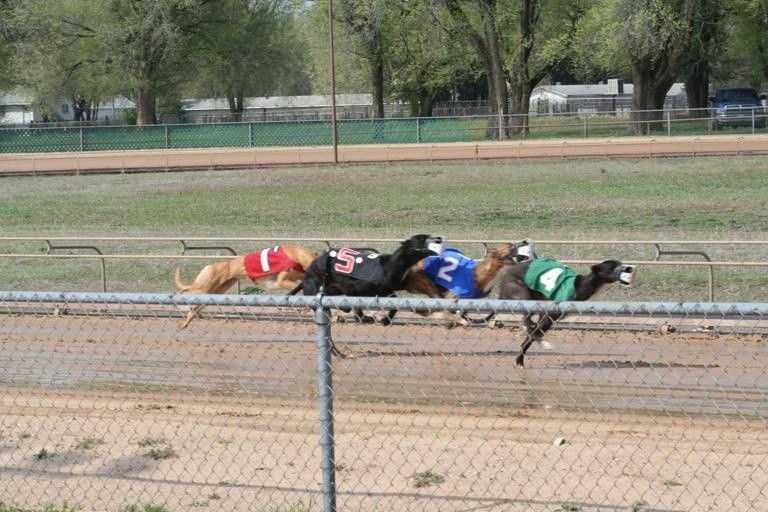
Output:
[286,234,443,359]
[450,258,633,370]
[174,243,328,330]
[388,240,529,329]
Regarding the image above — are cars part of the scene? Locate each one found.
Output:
[709,87,766,132]
